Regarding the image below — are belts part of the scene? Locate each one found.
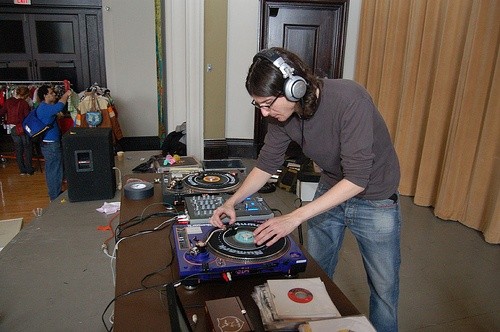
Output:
[389,194,398,203]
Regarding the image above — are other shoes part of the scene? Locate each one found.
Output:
[21,171,41,177]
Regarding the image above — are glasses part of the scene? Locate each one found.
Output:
[251,90,283,112]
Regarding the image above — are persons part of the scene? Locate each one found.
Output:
[35,85,72,201]
[209,46,402,332]
[0,86,37,176]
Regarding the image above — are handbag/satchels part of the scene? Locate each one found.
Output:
[86,112,102,126]
[16,122,25,137]
[22,103,56,143]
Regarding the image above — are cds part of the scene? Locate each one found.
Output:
[288,287,313,303]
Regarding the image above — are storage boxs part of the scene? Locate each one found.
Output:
[202,159,247,173]
[205,297,255,332]
[296,173,321,202]
[153,157,204,174]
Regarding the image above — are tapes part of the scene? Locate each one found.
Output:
[124,181,155,199]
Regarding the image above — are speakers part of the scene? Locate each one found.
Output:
[62,127,117,203]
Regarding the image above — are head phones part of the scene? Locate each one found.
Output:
[252,49,307,102]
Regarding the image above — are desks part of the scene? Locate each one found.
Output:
[114,173,362,332]
